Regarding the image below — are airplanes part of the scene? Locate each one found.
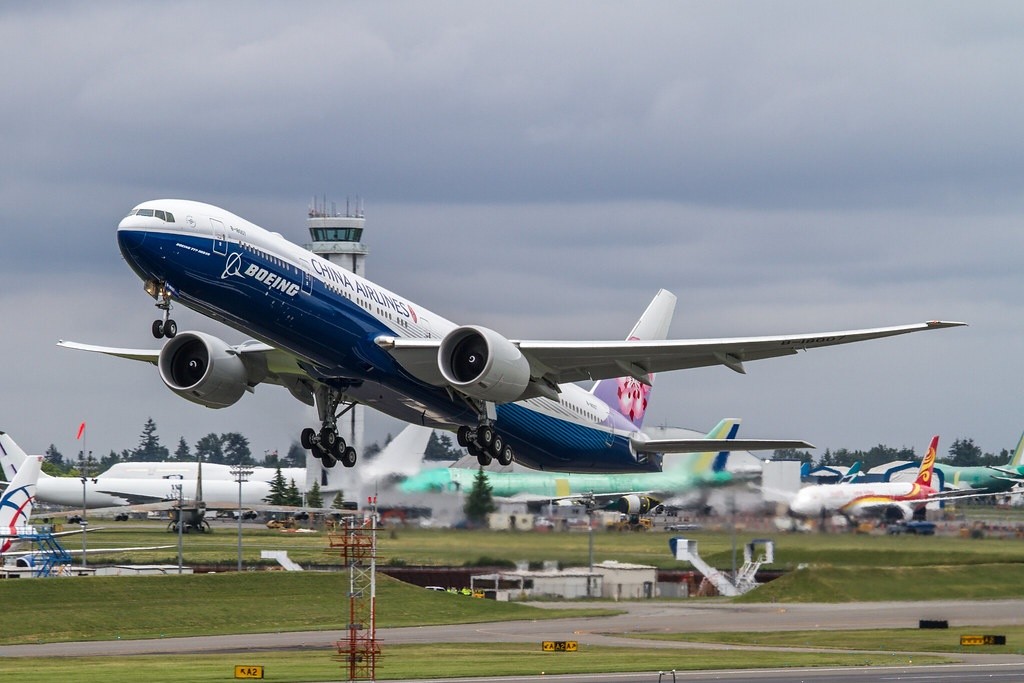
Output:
[0,417,1024,570]
[84,195,973,477]
